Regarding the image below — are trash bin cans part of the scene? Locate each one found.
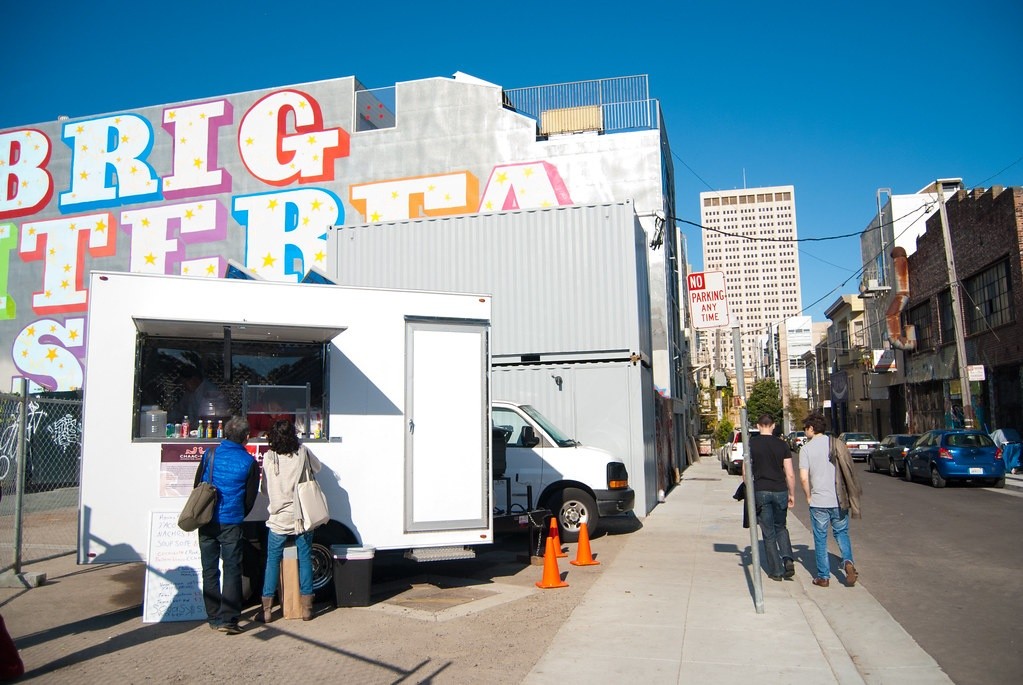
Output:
[331,545,376,608]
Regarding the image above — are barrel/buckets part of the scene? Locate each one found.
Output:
[140,410,167,437]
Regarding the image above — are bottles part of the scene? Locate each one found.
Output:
[217,420,223,438]
[166,416,190,438]
[197,420,203,438]
[314,421,321,439]
[206,420,213,438]
[302,432,306,438]
[310,433,314,438]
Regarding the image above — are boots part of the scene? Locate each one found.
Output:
[254,597,274,623]
[301,594,314,621]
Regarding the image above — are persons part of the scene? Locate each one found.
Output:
[742,415,796,581]
[166,367,228,425]
[799,413,858,586]
[247,387,293,438]
[254,418,321,624]
[194,417,260,633]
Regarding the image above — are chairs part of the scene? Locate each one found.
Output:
[847,438,855,441]
[962,438,972,445]
[863,438,871,441]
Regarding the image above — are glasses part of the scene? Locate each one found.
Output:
[803,426,810,431]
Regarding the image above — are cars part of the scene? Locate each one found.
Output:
[785,431,837,453]
[866,433,921,478]
[903,429,1007,488]
[838,432,880,462]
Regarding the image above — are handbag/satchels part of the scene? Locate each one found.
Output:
[281,559,301,619]
[298,481,330,532]
[178,484,217,531]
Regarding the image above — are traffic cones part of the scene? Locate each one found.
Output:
[543,516,568,558]
[568,522,600,567]
[535,537,570,589]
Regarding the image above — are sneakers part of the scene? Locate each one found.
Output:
[210,624,218,630]
[218,624,244,634]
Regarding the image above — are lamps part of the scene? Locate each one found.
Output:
[636,209,667,222]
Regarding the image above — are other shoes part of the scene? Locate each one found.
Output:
[768,574,782,581]
[845,562,856,586]
[813,577,829,587]
[785,560,795,577]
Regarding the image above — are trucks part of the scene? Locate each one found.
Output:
[77,270,637,562]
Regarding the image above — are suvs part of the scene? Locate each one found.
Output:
[720,429,761,476]
